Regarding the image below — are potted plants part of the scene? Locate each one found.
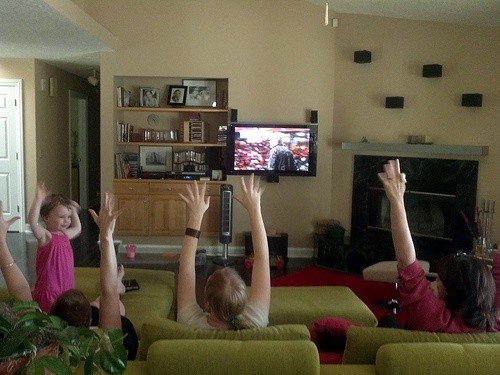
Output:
[0,296,128,375]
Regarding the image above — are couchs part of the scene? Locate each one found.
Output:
[0,266,500,375]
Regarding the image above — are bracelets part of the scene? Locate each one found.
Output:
[1,261,15,268]
[185,227,201,240]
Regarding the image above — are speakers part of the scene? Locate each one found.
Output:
[219,184,233,243]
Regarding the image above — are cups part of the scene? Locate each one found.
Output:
[422,136,433,144]
[125,245,136,260]
[487,248,498,259]
[408,135,418,144]
[212,170,223,180]
[473,238,488,256]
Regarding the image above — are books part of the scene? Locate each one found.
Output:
[115,86,134,109]
[115,152,129,178]
[116,121,132,142]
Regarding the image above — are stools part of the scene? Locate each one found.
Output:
[363,260,431,315]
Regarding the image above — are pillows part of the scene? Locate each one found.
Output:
[309,317,366,364]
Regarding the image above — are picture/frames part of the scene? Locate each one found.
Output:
[167,85,187,107]
[182,79,217,107]
[139,146,173,173]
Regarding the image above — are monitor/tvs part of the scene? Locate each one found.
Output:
[227,123,318,183]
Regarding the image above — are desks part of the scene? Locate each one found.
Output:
[314,232,345,269]
[245,285,378,328]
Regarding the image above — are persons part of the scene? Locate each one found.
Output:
[175,173,271,332]
[29,180,82,314]
[377,158,500,333]
[0,193,139,361]
[172,90,182,102]
[190,87,210,102]
[143,90,159,107]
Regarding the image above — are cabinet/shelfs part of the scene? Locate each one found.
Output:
[112,76,230,238]
[245,232,288,273]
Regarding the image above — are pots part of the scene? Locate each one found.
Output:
[327,224,345,239]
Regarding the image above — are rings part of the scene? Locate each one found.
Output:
[387,177,393,180]
